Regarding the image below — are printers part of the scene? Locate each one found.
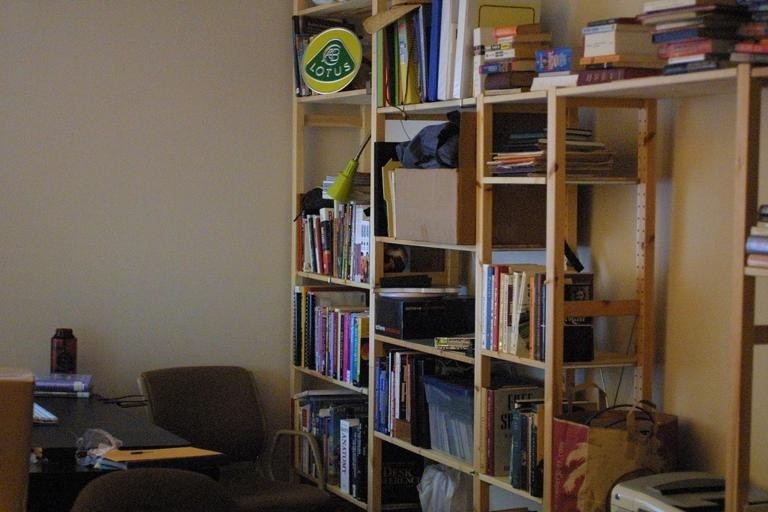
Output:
[611,470,768,512]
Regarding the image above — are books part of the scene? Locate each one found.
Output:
[34,373,95,391]
[33,389,91,398]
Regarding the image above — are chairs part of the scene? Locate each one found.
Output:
[137,364,309,512]
[70,467,238,512]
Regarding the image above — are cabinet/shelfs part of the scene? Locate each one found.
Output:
[289,0,768,512]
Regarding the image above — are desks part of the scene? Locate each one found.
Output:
[24,388,221,512]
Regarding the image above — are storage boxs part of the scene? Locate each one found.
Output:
[418,371,543,466]
[387,111,477,245]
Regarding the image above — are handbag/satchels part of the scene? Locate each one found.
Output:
[553,381,678,512]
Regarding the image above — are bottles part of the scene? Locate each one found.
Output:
[50,328,77,374]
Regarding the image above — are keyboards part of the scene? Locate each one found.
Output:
[32,401,59,425]
